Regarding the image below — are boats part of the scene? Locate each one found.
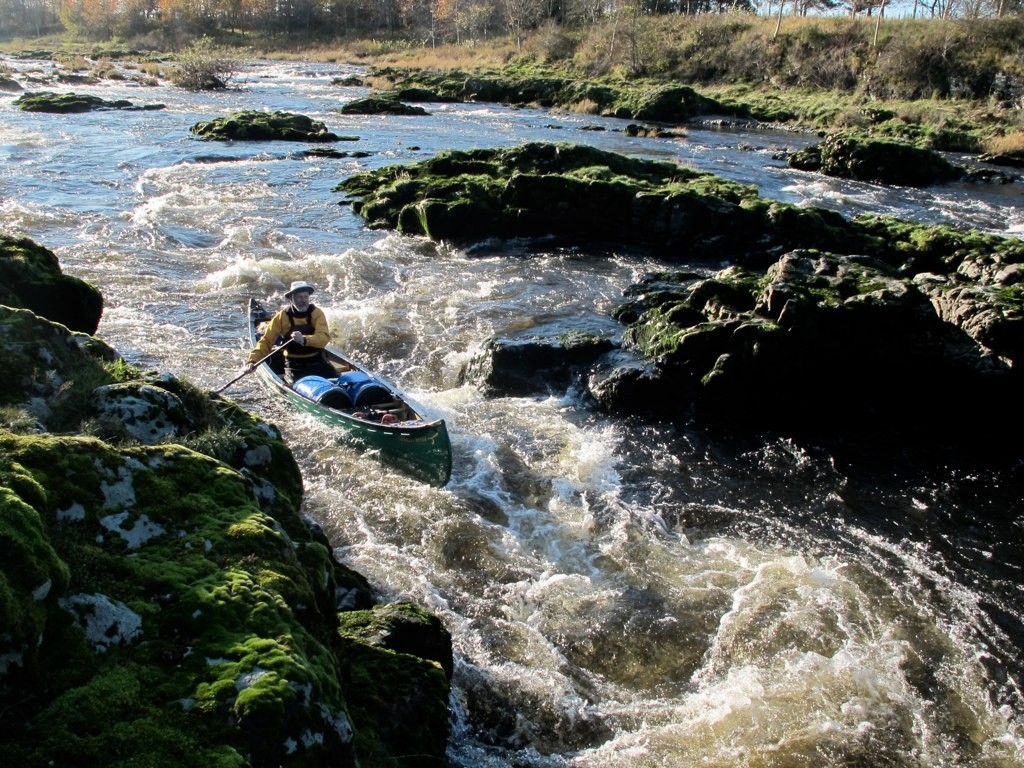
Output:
[247,298,452,478]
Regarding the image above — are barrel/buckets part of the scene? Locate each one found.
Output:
[334,371,397,409]
[291,375,353,411]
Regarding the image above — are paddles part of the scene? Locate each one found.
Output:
[213,338,294,394]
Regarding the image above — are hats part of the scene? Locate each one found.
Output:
[284,281,315,298]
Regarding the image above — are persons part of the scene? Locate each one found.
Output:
[243,281,338,385]
